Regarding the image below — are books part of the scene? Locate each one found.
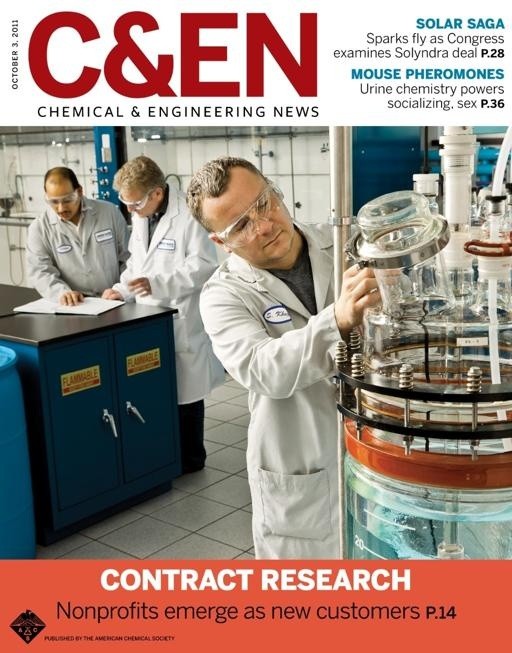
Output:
[14,294,125,316]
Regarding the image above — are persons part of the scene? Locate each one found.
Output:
[187,155,409,560]
[112,156,230,474]
[23,167,131,305]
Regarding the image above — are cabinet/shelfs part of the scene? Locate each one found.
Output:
[0,298,182,545]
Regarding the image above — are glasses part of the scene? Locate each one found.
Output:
[216,177,285,248]
[118,188,154,210]
[44,188,78,207]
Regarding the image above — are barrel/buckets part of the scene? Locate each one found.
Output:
[0,345,38,559]
